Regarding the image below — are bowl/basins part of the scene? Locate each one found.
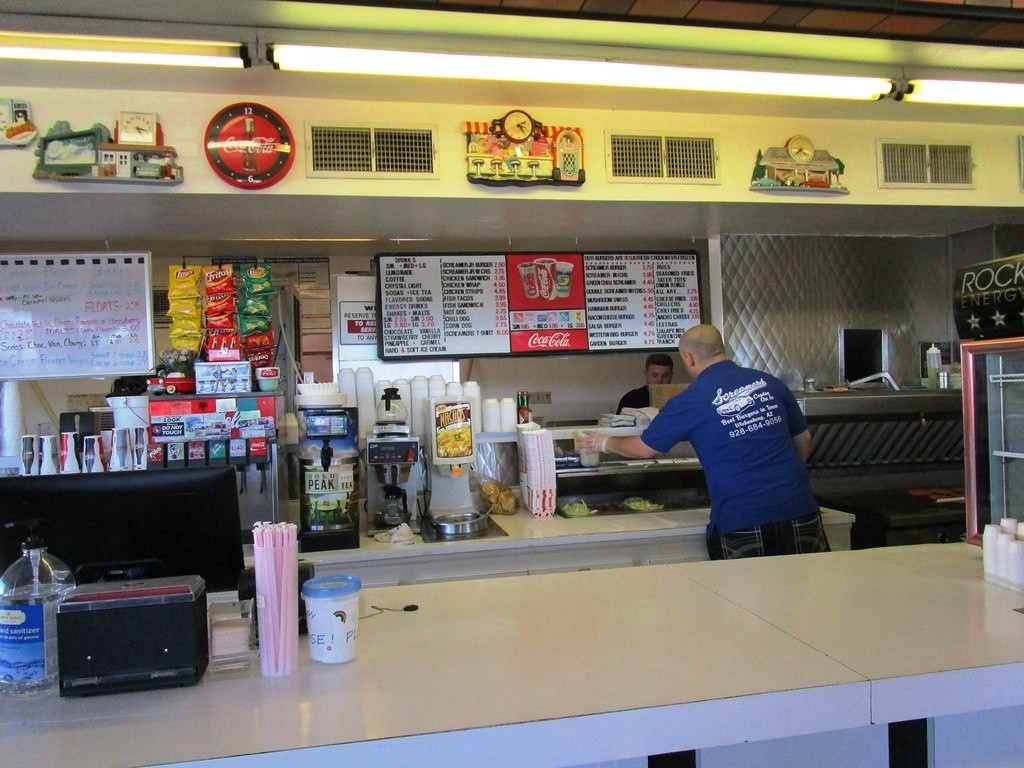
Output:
[297,382,339,394]
[982,518,1024,582]
[295,394,346,407]
[259,378,279,392]
[338,367,517,438]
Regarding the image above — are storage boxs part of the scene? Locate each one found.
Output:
[57,575,210,698]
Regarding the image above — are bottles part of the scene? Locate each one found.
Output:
[73,434,79,463]
[60,434,68,471]
[116,430,127,467]
[39,438,44,474]
[84,438,95,472]
[135,428,144,464]
[936,372,949,388]
[0,536,76,698]
[805,378,816,391]
[517,391,532,423]
[97,437,106,472]
[50,437,60,473]
[22,437,34,474]
[926,342,941,387]
[242,118,257,171]
[518,391,522,407]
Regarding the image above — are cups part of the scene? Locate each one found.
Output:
[82,435,107,473]
[19,434,39,475]
[518,262,540,299]
[109,428,134,471]
[101,431,112,449]
[534,258,557,300]
[134,426,148,469]
[303,372,314,384]
[59,432,79,473]
[300,574,362,664]
[38,435,59,475]
[556,262,574,297]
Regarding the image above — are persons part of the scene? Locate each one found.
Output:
[574,324,832,560]
[615,354,676,415]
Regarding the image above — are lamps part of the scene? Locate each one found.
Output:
[868,80,915,102]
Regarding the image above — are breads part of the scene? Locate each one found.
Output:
[482,482,517,515]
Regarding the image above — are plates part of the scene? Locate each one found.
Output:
[804,391,822,393]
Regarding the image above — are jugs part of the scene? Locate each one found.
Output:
[375,485,408,528]
[376,387,408,422]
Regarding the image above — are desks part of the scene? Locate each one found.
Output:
[0,541,1024,768]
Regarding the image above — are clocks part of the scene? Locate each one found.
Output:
[204,102,295,189]
[787,136,815,163]
[116,111,157,147]
[502,111,534,143]
